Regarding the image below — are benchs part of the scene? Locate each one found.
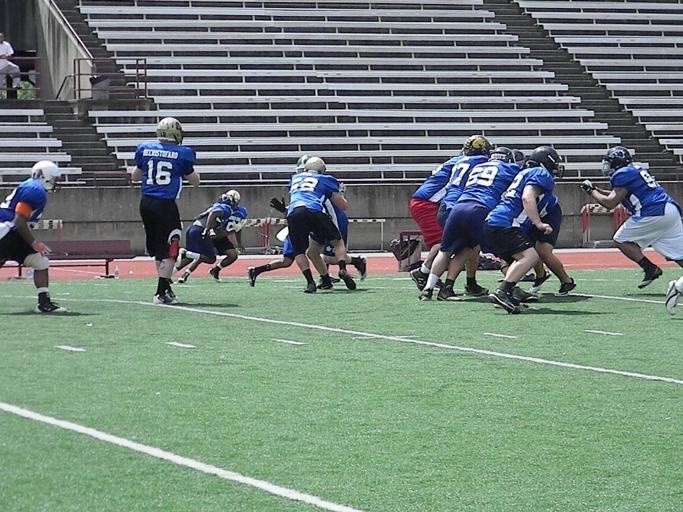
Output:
[0,0,683,186]
[1,240,136,279]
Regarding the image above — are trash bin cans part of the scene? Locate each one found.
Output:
[397,239,422,269]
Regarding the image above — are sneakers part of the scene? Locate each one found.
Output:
[519,269,550,292]
[153,286,179,304]
[638,267,663,288]
[488,285,540,314]
[304,256,366,293]
[208,266,221,279]
[175,248,187,268]
[554,277,577,297]
[249,267,257,287]
[178,272,189,283]
[34,302,67,314]
[410,266,489,301]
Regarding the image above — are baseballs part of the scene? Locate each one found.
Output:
[130,271,133,274]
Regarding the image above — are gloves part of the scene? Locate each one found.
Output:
[31,239,53,256]
[579,179,598,196]
[202,227,216,240]
[270,197,286,213]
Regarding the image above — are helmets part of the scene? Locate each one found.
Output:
[461,135,564,177]
[601,146,632,176]
[295,153,326,175]
[221,189,241,212]
[31,160,62,192]
[156,117,183,145]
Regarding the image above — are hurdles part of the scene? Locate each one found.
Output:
[580,203,630,248]
[231,215,290,254]
[346,218,388,253]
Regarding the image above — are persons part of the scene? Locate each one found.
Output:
[579,146,683,288]
[0,32,23,89]
[176,189,247,284]
[248,155,368,293]
[131,116,200,306]
[406,137,576,313]
[0,160,68,314]
